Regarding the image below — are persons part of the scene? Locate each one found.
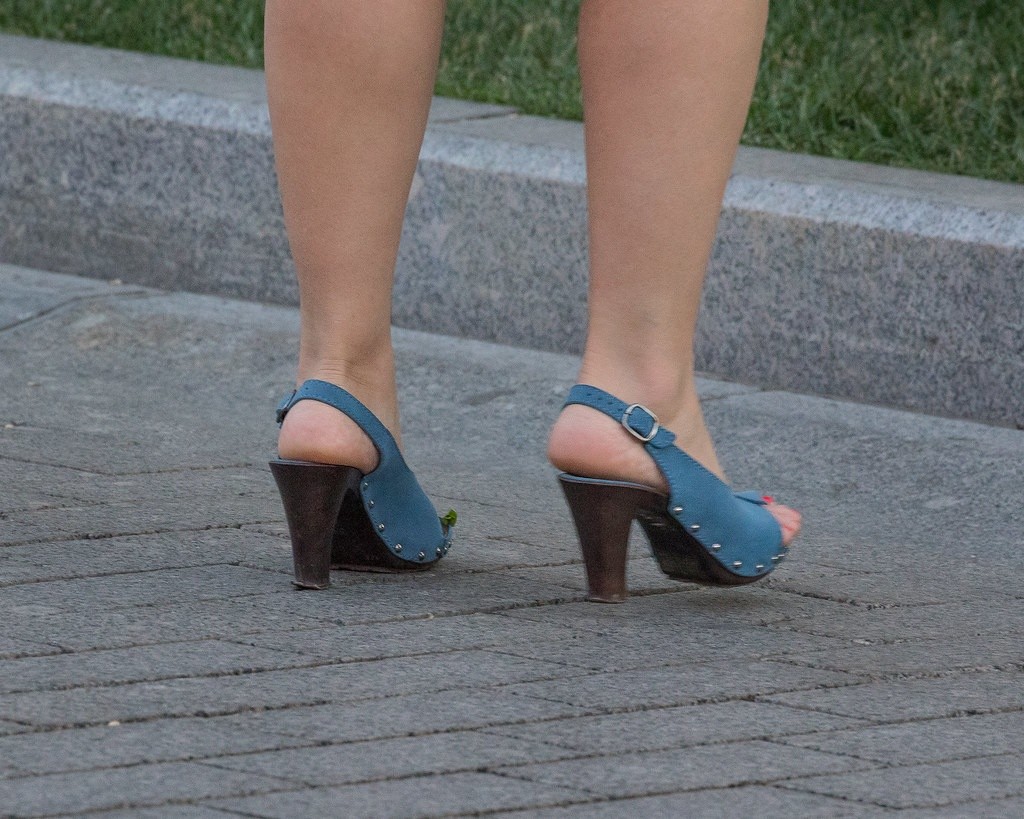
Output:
[264,0,803,602]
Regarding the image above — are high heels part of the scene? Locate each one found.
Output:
[267,380,454,588]
[557,384,797,604]
